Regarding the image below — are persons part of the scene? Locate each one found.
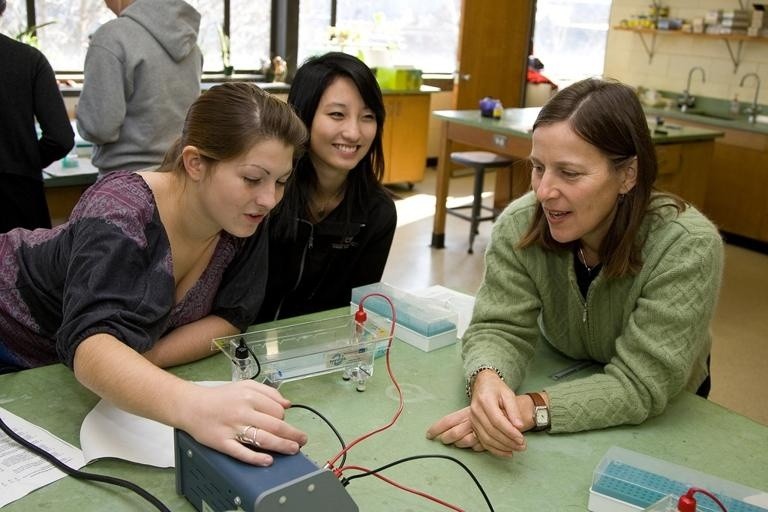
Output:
[254,51,397,327]
[422,77,727,458]
[0,81,310,466]
[72,1,204,179]
[0,1,76,231]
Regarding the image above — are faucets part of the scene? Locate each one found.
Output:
[739,73,760,108]
[684,66,705,98]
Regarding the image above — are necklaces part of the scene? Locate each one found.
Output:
[309,186,342,219]
[578,247,600,272]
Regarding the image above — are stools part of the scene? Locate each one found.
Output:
[448,149,522,255]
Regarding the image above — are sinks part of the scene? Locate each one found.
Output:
[689,110,738,121]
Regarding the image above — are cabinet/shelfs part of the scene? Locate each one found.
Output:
[370,93,435,190]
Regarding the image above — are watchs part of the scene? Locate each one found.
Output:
[525,390,551,434]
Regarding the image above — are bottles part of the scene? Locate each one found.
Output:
[479,94,503,120]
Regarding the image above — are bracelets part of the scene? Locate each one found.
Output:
[465,364,504,401]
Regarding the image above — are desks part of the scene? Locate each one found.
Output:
[0,287,768,512]
[431,103,727,250]
[38,148,109,234]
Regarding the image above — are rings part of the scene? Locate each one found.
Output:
[237,423,261,448]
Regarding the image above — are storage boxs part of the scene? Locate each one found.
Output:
[375,66,423,92]
